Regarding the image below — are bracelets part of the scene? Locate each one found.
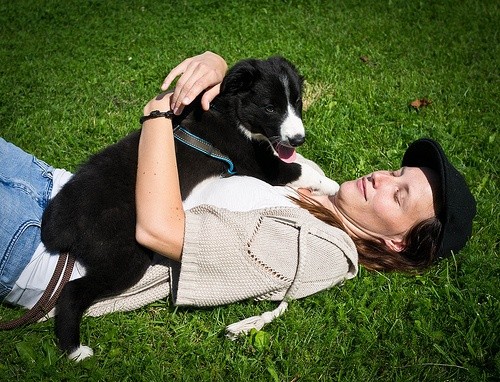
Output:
[139,110,174,124]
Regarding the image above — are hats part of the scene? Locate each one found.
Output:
[402,138,477,264]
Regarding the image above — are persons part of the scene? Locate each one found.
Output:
[0,51,476,341]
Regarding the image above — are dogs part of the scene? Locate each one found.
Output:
[40,55,305,364]
